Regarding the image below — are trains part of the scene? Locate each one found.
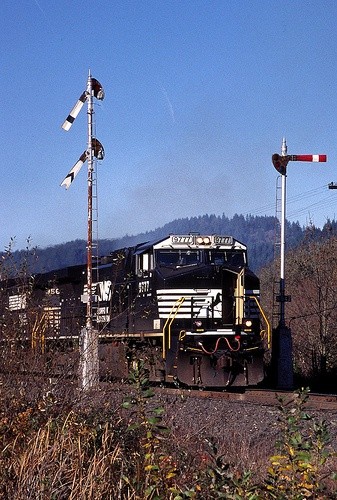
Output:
[0,231,268,393]
